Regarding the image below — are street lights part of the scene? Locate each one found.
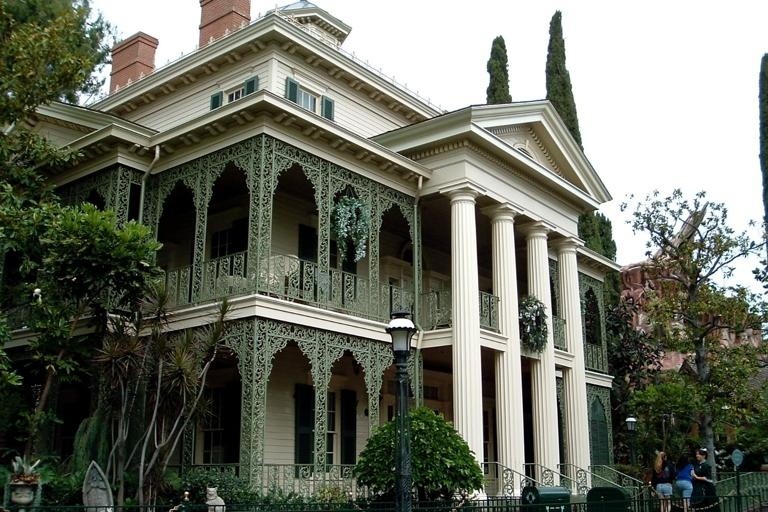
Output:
[384,310,420,510]
[625,413,639,485]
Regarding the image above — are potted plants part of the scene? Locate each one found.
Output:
[8,455,40,504]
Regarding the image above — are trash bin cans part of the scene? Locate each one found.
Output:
[522,487,572,512]
[587,487,631,511]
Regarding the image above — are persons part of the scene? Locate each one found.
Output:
[651,452,678,512]
[674,454,706,511]
[689,447,721,511]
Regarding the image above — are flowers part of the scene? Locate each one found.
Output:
[332,195,372,263]
[518,293,547,361]
